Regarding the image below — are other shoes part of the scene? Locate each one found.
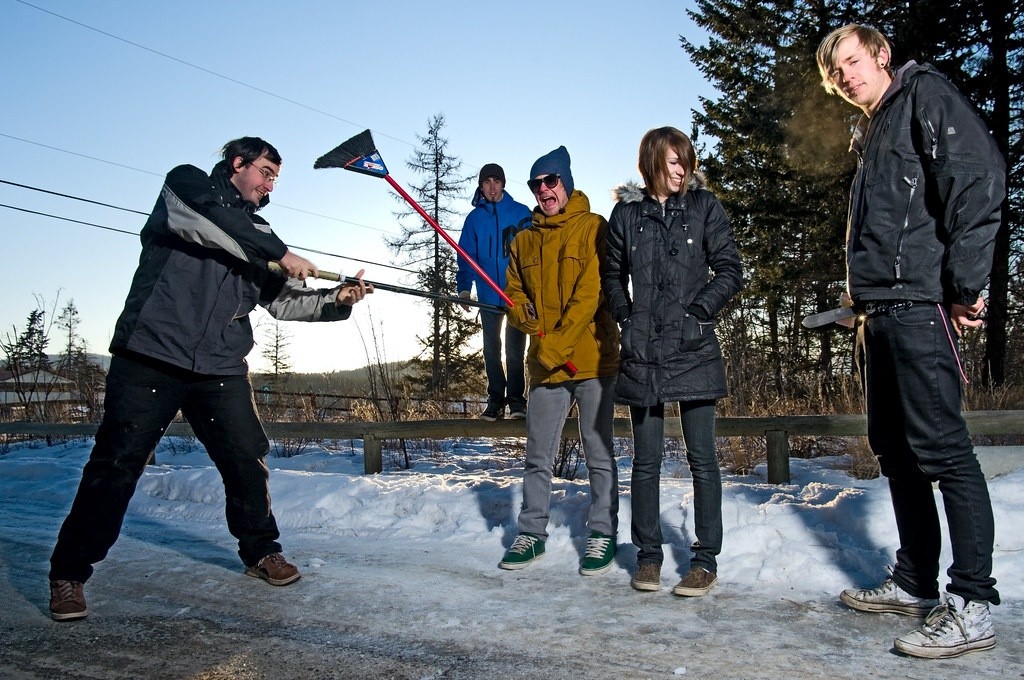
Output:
[674,566,716,596]
[631,561,661,591]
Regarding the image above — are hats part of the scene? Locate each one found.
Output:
[479,164,505,188]
[531,146,573,196]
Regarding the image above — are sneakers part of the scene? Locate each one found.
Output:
[580,530,618,576]
[498,533,545,570]
[480,407,504,422]
[840,564,940,617]
[245,553,301,585]
[893,592,997,659]
[510,407,526,420]
[48,580,88,620]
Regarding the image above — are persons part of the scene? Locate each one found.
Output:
[499,146,621,573]
[455,163,534,422]
[598,126,745,597]
[815,24,1006,660]
[48,137,373,619]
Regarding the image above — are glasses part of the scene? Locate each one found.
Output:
[249,161,278,183]
[527,174,561,193]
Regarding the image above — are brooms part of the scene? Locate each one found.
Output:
[312,129,579,376]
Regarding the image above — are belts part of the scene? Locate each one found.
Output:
[802,300,912,329]
